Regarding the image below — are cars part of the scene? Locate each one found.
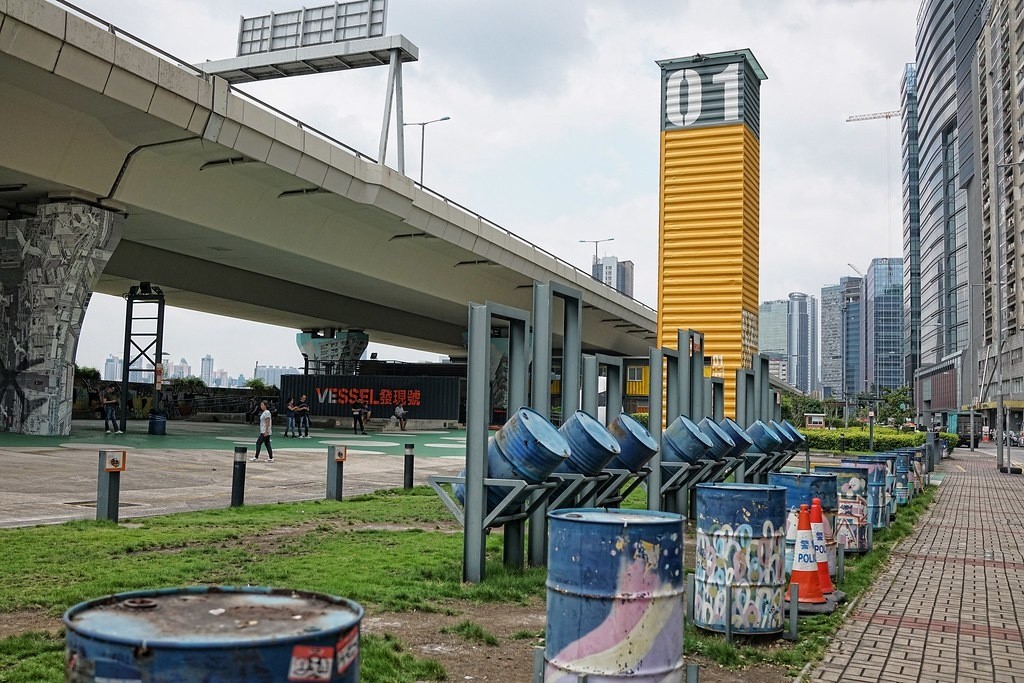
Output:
[1001,430,1024,447]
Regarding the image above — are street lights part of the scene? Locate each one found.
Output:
[579,237,615,278]
[400,116,451,190]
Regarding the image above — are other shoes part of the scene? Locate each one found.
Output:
[305,435,312,438]
[401,428,407,431]
[298,436,304,438]
[284,436,289,439]
[249,458,259,462]
[361,432,367,434]
[264,458,274,462]
[367,419,371,422]
[292,437,297,439]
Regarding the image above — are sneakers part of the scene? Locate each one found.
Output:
[106,430,111,433]
[115,430,123,433]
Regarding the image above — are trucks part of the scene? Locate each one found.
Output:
[944,411,984,449]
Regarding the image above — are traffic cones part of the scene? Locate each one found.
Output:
[777,504,836,617]
[808,498,847,603]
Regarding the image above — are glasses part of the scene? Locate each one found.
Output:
[111,388,115,390]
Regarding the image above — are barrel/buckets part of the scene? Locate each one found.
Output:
[638,414,713,487]
[815,447,926,554]
[548,409,621,507]
[694,483,788,634]
[697,417,736,462]
[452,406,571,528]
[596,412,660,500]
[747,418,806,472]
[543,507,687,683]
[719,417,754,459]
[767,473,838,584]
[65,585,365,683]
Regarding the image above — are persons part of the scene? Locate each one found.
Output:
[246,398,260,424]
[103,384,123,433]
[250,400,274,462]
[352,397,371,435]
[284,394,311,438]
[395,402,407,431]
